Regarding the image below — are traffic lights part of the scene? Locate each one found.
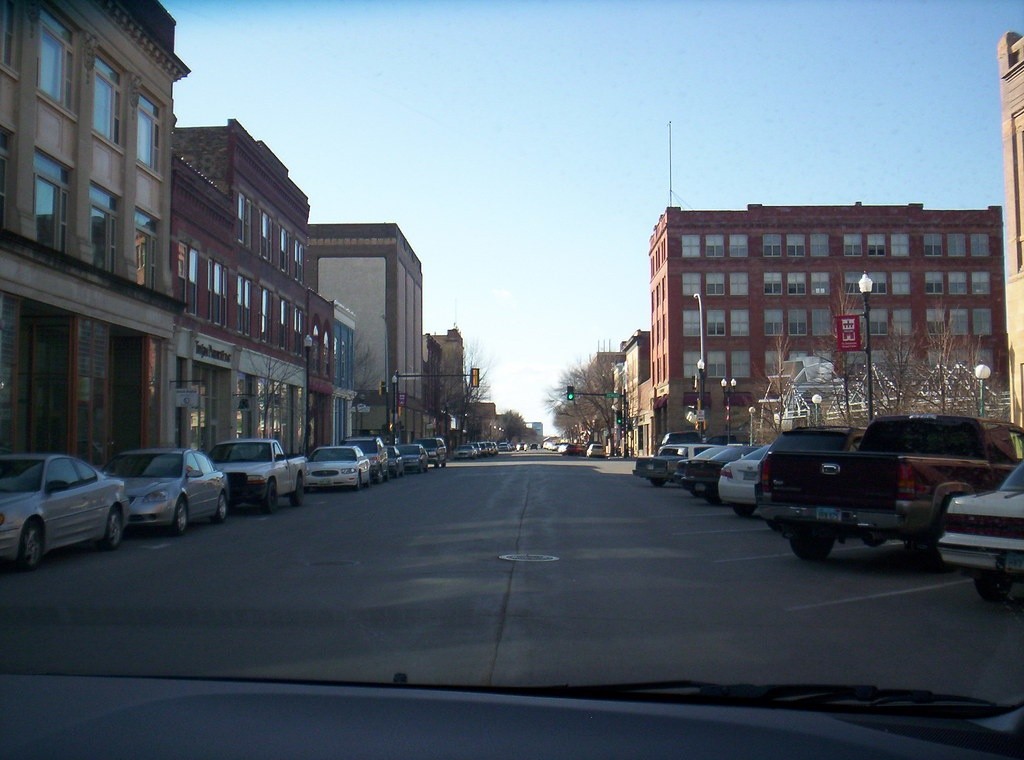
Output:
[616,410,622,424]
[567,386,574,400]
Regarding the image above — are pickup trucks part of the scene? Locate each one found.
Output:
[207,438,308,514]
[751,414,1024,562]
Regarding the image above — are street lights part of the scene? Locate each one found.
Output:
[812,394,823,428]
[303,334,313,454]
[693,293,705,360]
[858,270,874,426]
[696,358,705,438]
[391,375,398,444]
[720,378,737,444]
[974,364,991,419]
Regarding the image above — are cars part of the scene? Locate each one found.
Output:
[385,444,428,478]
[304,446,371,491]
[453,442,527,459]
[529,442,606,457]
[101,447,230,534]
[935,460,1024,601]
[0,453,133,572]
[632,430,772,517]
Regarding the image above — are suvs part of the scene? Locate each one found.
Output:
[412,437,447,468]
[753,425,867,509]
[339,434,389,484]
